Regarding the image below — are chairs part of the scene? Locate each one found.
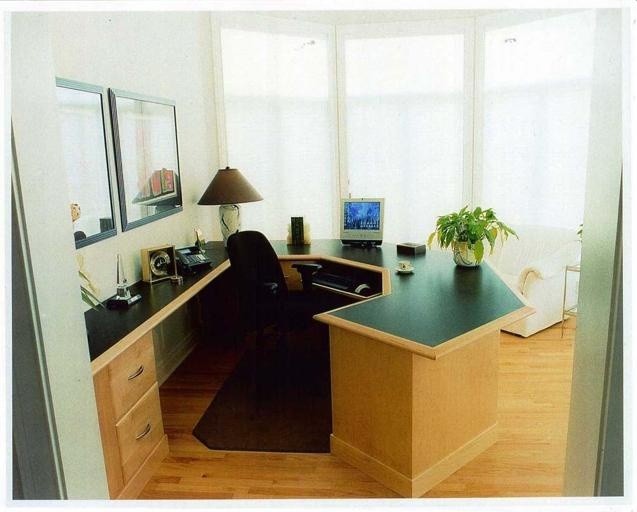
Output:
[226,231,326,401]
[482,223,582,339]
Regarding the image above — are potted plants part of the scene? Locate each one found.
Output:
[428,204,520,269]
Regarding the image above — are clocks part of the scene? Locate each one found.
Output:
[140,243,178,285]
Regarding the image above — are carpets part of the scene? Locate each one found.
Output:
[191,350,333,454]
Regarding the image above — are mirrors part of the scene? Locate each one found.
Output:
[55,76,117,250]
[108,86,183,232]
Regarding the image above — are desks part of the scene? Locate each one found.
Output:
[260,235,536,498]
[84,240,230,499]
[560,260,581,341]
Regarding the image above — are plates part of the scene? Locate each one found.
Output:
[394,266,415,273]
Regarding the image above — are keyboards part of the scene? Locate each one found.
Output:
[310,271,354,291]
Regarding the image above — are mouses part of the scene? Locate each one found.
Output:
[355,282,371,295]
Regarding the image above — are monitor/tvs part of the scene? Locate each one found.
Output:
[340,198,384,246]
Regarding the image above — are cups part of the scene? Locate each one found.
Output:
[398,260,411,270]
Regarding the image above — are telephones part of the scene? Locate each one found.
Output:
[175,246,213,274]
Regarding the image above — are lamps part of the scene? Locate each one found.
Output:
[198,165,264,248]
